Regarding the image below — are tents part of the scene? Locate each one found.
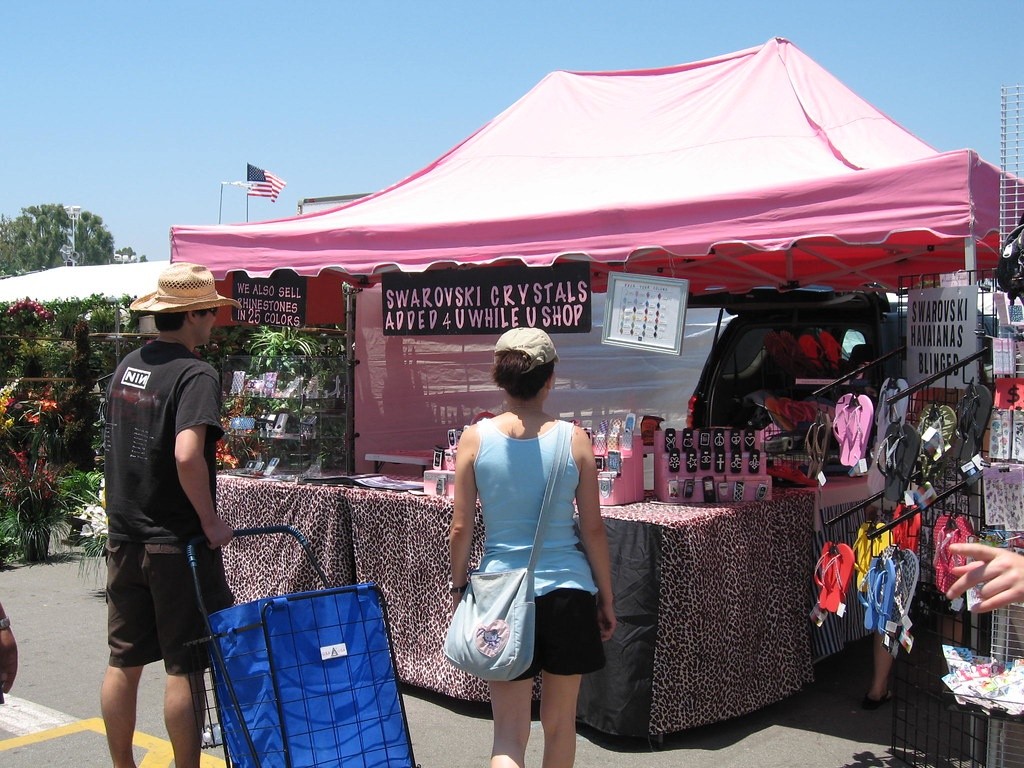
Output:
[170,36,1024,768]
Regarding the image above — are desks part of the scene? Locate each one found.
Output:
[212,470,903,749]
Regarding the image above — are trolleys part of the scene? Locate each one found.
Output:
[183,525,422,768]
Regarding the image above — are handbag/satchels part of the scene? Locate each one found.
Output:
[442,567,535,681]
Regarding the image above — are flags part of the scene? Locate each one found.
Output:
[247,163,285,204]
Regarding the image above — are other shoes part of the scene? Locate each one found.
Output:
[862,688,893,710]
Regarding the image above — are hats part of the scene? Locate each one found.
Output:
[494,327,559,375]
[130,263,242,312]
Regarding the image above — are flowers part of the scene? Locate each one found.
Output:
[0,442,76,560]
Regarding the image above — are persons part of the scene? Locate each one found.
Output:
[0,603,18,705]
[946,543,1024,614]
[101,261,242,768]
[860,624,896,710]
[448,328,616,768]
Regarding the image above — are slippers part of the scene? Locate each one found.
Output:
[857,558,895,634]
[877,422,919,503]
[875,377,909,444]
[833,393,874,467]
[881,544,919,626]
[917,405,957,480]
[891,503,921,554]
[952,385,992,461]
[814,541,854,613]
[803,411,829,480]
[933,513,973,594]
[852,521,893,592]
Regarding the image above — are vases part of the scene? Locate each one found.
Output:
[24,529,50,561]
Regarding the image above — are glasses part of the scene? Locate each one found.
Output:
[207,308,218,315]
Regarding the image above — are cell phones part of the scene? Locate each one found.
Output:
[249,457,280,477]
[432,425,471,497]
[582,412,637,477]
[668,474,768,504]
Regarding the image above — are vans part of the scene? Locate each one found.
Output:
[685,281,995,474]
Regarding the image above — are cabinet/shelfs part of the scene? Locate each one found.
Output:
[214,353,353,475]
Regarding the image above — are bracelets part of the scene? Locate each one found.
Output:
[0,616,10,630]
[450,583,468,593]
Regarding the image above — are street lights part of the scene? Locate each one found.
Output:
[217,180,255,225]
[63,205,81,250]
[112,251,138,368]
